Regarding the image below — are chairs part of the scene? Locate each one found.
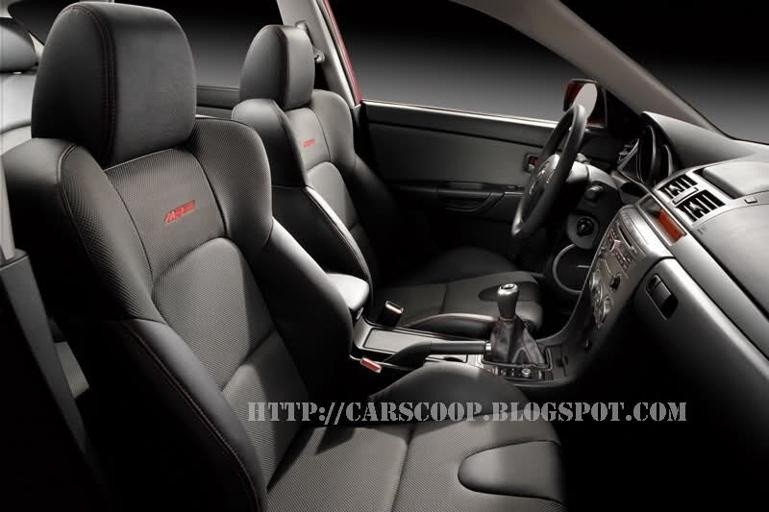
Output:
[230,24,544,338]
[0,2,564,512]
[1,17,39,256]
